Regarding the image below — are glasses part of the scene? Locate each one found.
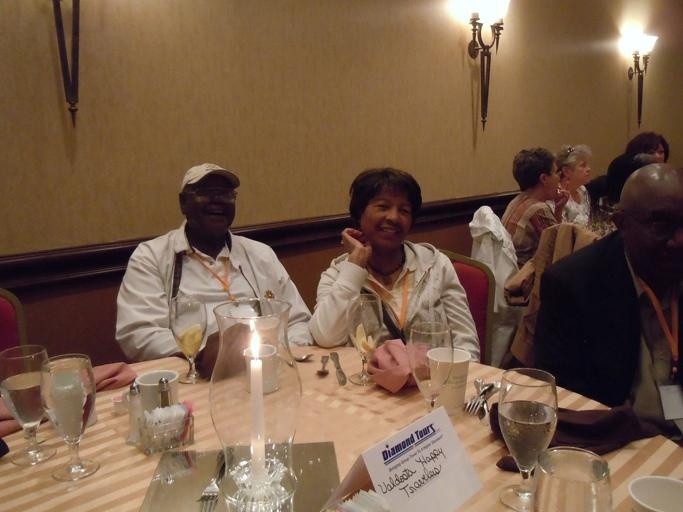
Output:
[188,188,238,199]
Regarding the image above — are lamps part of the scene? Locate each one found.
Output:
[449,1,511,133]
[621,21,656,129]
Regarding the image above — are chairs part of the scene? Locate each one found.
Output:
[0,289,33,387]
[431,245,497,370]
[526,223,597,365]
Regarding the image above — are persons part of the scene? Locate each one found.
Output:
[532,163,683,449]
[622,130,669,168]
[311,166,481,369]
[496,145,569,271]
[545,142,592,229]
[112,162,315,361]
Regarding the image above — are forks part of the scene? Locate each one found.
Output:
[197,447,234,502]
[464,397,485,416]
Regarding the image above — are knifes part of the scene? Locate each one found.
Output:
[330,352,348,385]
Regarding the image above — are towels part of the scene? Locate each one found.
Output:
[1,360,138,438]
[194,322,251,379]
[490,397,658,475]
[366,338,429,392]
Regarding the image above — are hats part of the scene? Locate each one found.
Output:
[180,162,240,191]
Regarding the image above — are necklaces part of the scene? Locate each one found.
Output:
[365,250,406,285]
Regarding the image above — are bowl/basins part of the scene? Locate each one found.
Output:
[629,475,683,512]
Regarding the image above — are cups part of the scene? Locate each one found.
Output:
[169,294,208,385]
[136,370,180,407]
[498,367,557,511]
[0,344,57,466]
[40,353,101,481]
[530,446,612,512]
[408,321,453,413]
[425,346,470,416]
[244,345,283,395]
[345,294,384,387]
